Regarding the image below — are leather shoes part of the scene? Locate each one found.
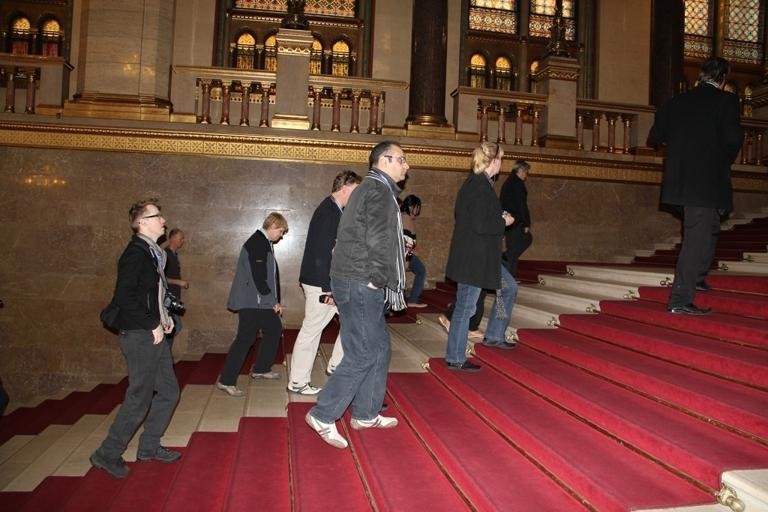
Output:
[696,281,708,290]
[667,303,712,315]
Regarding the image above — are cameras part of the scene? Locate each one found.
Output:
[163,292,185,316]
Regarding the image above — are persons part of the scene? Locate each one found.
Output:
[646,57,744,316]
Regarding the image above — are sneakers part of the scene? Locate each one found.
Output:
[447,360,480,371]
[137,446,180,463]
[90,454,130,480]
[305,413,348,448]
[350,414,398,430]
[217,371,333,397]
[483,337,516,349]
